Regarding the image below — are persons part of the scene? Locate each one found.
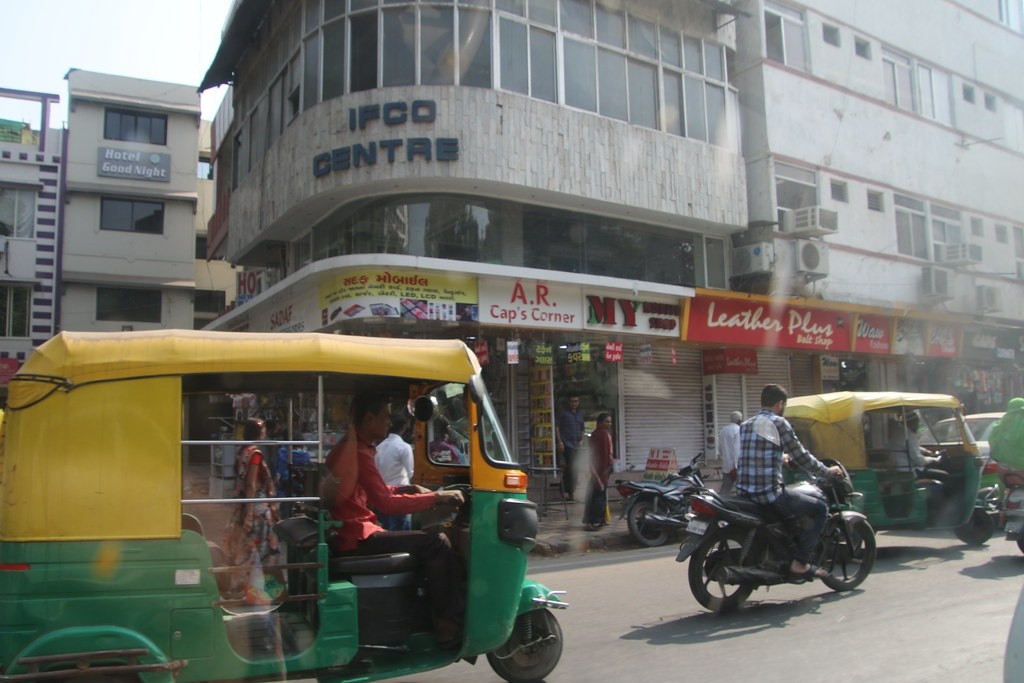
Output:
[222,420,289,607]
[263,418,312,519]
[555,393,585,501]
[718,411,743,497]
[583,413,614,531]
[427,413,464,465]
[374,413,415,531]
[735,382,844,580]
[988,398,1024,489]
[324,389,465,650]
[900,410,953,501]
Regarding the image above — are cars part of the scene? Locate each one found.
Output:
[924,412,1011,510]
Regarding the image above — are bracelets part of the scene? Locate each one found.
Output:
[246,516,253,522]
[434,488,444,507]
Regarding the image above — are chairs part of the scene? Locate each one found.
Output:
[304,468,422,579]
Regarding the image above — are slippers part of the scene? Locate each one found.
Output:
[790,564,830,578]
[441,635,463,650]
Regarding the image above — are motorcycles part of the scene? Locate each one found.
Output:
[611,451,716,548]
[786,391,997,550]
[0,331,570,682]
[674,458,877,615]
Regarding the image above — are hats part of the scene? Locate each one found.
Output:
[905,411,918,421]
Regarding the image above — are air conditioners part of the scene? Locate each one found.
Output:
[793,207,837,234]
[921,266,961,299]
[942,240,983,263]
[731,240,776,275]
[790,239,829,277]
[975,286,1004,312]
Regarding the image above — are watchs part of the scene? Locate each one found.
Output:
[558,441,563,444]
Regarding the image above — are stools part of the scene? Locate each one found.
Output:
[527,467,571,524]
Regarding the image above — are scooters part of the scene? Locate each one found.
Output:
[997,466,1024,553]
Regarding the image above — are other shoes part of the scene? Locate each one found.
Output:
[585,525,597,531]
